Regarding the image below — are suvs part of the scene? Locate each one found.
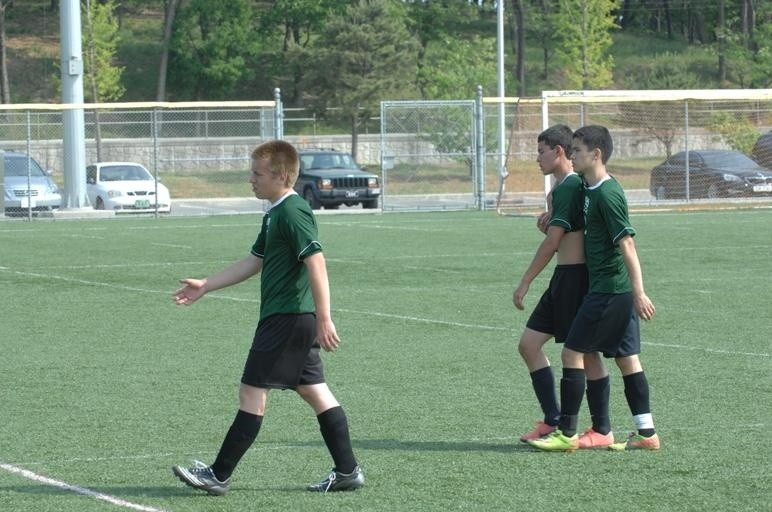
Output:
[293,147,381,211]
[750,128,772,169]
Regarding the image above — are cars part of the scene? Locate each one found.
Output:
[647,148,772,200]
[85,162,172,216]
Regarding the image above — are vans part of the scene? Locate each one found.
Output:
[1,149,61,217]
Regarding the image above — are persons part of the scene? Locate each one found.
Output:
[169,140,367,498]
[525,124,661,454]
[512,123,617,449]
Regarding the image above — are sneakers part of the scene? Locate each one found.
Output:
[579,428,614,449]
[526,429,579,452]
[172,460,231,495]
[608,430,660,450]
[307,464,365,491]
[520,419,557,445]
